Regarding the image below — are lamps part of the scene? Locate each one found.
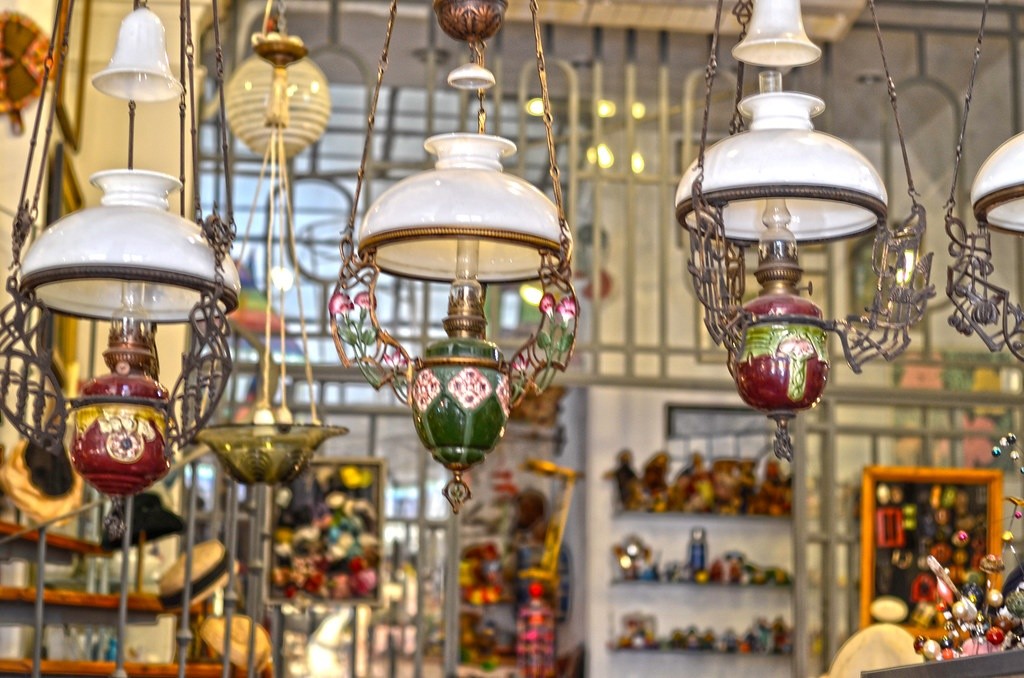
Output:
[674,0,938,466]
[0,0,238,544]
[328,0,583,518]
[194,0,354,488]
[942,0,1024,367]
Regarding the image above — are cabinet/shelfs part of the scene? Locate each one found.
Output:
[0,520,238,678]
